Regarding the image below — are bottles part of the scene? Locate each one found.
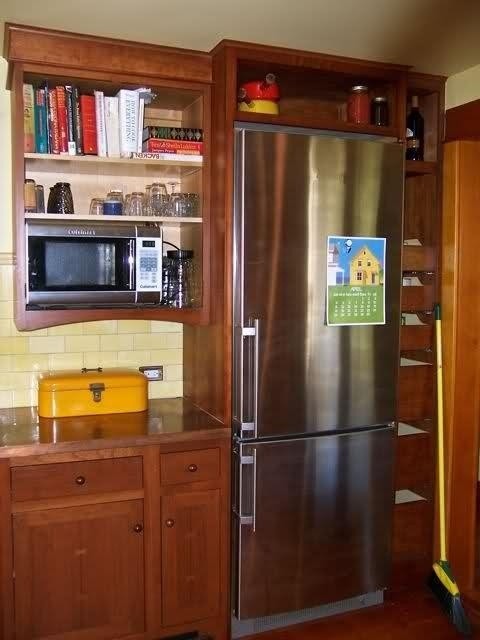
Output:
[406,93,423,160]
[163,250,194,308]
[349,85,388,127]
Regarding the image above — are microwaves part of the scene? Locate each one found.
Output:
[25,223,164,308]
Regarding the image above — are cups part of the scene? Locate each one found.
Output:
[24,180,199,217]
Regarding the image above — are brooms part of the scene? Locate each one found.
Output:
[424,304,471,635]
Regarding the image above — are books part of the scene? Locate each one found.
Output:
[17,77,206,165]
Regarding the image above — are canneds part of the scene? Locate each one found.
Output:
[347,86,371,125]
[371,97,388,125]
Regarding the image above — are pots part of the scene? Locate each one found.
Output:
[238,73,280,114]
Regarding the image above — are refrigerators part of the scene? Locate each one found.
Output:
[232,123,399,638]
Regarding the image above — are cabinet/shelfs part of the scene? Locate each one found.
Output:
[388,72,449,597]
[0,435,145,639]
[3,23,212,332]
[159,428,231,637]
